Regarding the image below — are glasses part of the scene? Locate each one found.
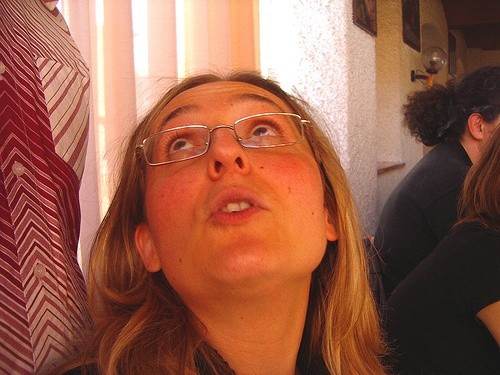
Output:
[134,113,322,169]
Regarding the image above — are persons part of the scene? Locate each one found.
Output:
[40,62,404,374]
[361,66,500,313]
[376,118,500,375]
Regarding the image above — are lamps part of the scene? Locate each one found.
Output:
[421,45,448,75]
